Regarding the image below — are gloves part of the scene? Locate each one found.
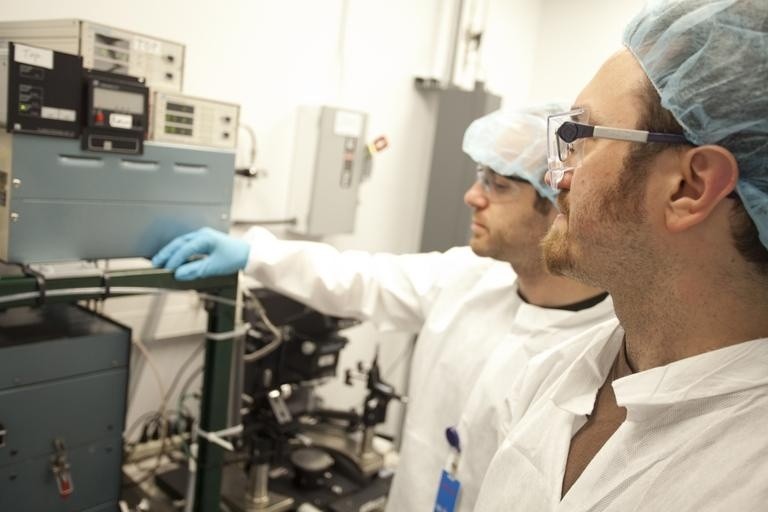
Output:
[150,225,251,283]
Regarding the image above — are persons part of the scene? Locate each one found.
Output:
[147,96,617,512]
[427,0,767,511]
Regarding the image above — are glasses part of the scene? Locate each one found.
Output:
[545,106,689,197]
[474,164,536,205]
